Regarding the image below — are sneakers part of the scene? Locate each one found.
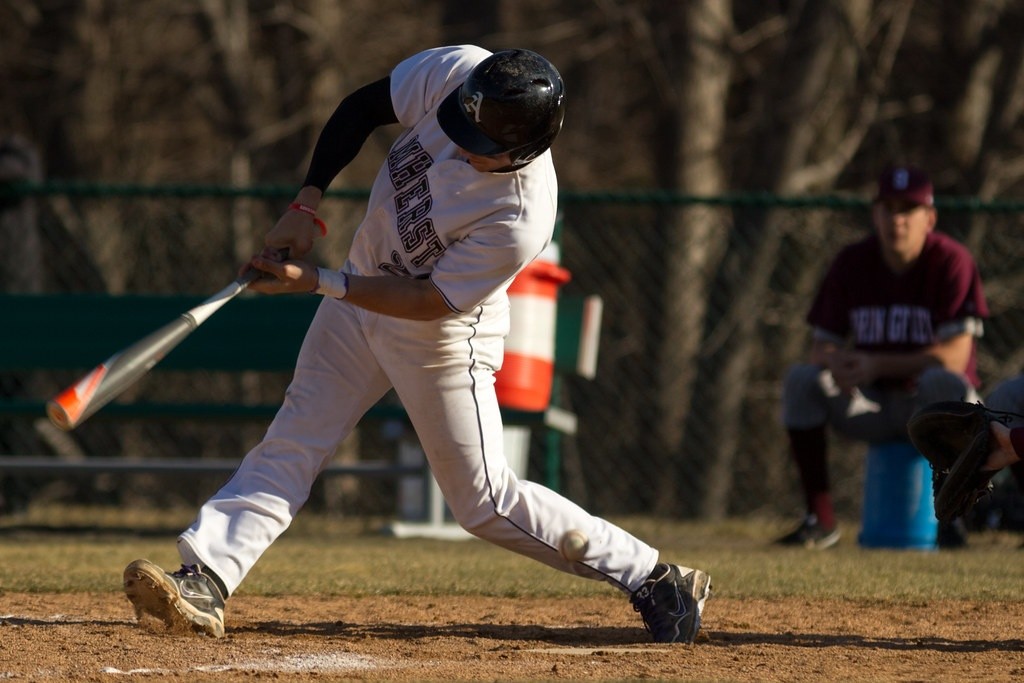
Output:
[627,561,714,644]
[122,557,227,640]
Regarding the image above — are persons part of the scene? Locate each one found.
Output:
[770,173,1024,552]
[124,44,711,645]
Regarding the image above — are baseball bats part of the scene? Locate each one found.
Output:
[45,245,294,433]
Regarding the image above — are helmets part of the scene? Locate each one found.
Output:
[435,47,568,168]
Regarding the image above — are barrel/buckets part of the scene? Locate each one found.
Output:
[495,260,571,415]
[856,445,936,548]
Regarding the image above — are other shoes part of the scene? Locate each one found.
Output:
[933,514,973,552]
[770,507,845,553]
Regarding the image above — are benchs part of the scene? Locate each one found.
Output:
[0,293,602,539]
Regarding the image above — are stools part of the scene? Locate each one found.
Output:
[857,443,940,551]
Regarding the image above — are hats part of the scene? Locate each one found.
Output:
[875,163,936,213]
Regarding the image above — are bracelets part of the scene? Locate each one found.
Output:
[288,204,327,239]
[308,267,349,300]
